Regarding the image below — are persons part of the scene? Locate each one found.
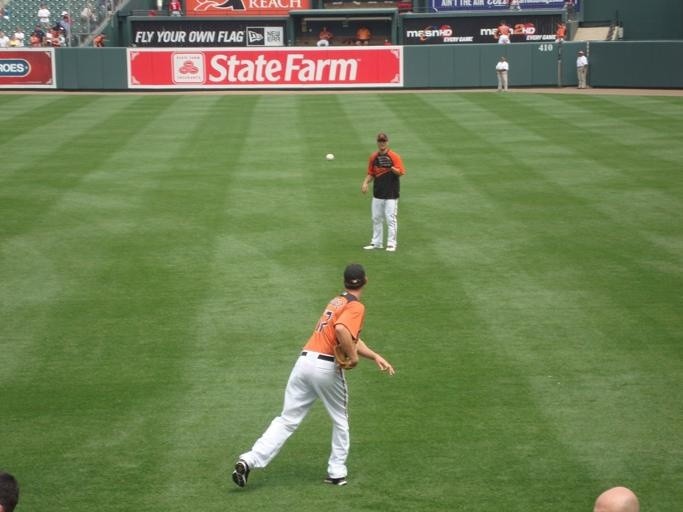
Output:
[576,50,588,89]
[0,470,19,512]
[592,486,640,512]
[316,26,333,47]
[0,1,107,48]
[495,57,509,92]
[168,0,183,17]
[361,134,405,252]
[494,20,512,43]
[355,25,371,46]
[231,264,395,488]
[554,21,567,44]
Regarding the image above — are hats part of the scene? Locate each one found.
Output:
[377,133,388,142]
[343,264,365,284]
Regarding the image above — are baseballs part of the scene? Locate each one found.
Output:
[326,153,334,160]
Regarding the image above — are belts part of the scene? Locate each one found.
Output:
[301,352,334,362]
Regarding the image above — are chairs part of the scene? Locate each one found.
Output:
[0,0,101,47]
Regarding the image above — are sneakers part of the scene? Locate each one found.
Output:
[323,476,347,486]
[231,460,249,487]
[362,243,383,250]
[385,246,395,252]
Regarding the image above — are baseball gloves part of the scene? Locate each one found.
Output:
[376,156,392,167]
[334,342,357,369]
